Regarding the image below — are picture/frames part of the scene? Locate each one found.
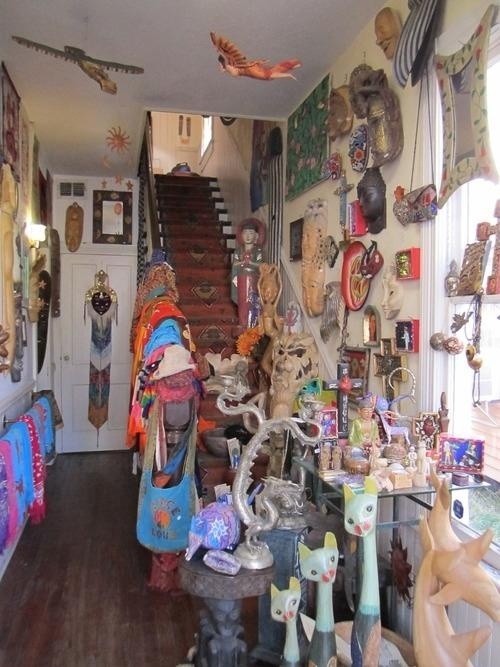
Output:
[348,347,370,404]
[316,410,339,440]
[289,218,304,261]
[93,189,132,244]
[435,435,484,474]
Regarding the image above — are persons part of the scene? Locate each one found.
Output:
[374,5,401,59]
[348,399,380,449]
[231,223,265,305]
[357,166,387,232]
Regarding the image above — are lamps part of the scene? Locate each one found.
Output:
[24,223,46,249]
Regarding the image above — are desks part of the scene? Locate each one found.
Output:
[292,456,491,604]
[177,553,274,667]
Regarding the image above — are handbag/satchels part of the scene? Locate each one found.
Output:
[136,475,201,554]
